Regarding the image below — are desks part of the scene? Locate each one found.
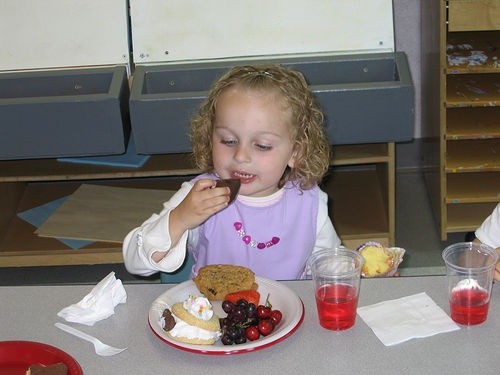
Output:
[0,273,500,375]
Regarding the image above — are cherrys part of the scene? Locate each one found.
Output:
[245,293,282,341]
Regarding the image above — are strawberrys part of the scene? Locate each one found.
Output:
[225,289,260,307]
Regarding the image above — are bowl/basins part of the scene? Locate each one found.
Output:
[148,275,305,355]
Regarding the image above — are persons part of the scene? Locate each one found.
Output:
[457,199,500,282]
[122,64,400,280]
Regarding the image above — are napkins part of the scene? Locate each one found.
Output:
[57,271,128,326]
[356,292,461,347]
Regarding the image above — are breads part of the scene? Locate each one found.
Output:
[25,362,68,375]
[210,179,241,204]
[359,245,399,278]
[193,265,255,300]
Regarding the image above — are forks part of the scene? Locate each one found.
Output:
[55,322,128,356]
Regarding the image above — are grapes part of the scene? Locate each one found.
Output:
[219,298,261,345]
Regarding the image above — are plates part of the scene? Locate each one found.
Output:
[0,340,84,375]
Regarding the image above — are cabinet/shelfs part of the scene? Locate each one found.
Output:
[438,0,500,240]
[0,142,396,268]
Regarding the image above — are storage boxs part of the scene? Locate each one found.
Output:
[128,49,416,155]
[0,66,130,160]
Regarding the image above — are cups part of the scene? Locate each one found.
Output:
[308,247,365,331]
[442,241,498,326]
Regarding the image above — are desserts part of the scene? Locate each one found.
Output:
[158,294,221,346]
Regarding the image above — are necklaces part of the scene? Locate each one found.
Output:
[233,191,286,249]
